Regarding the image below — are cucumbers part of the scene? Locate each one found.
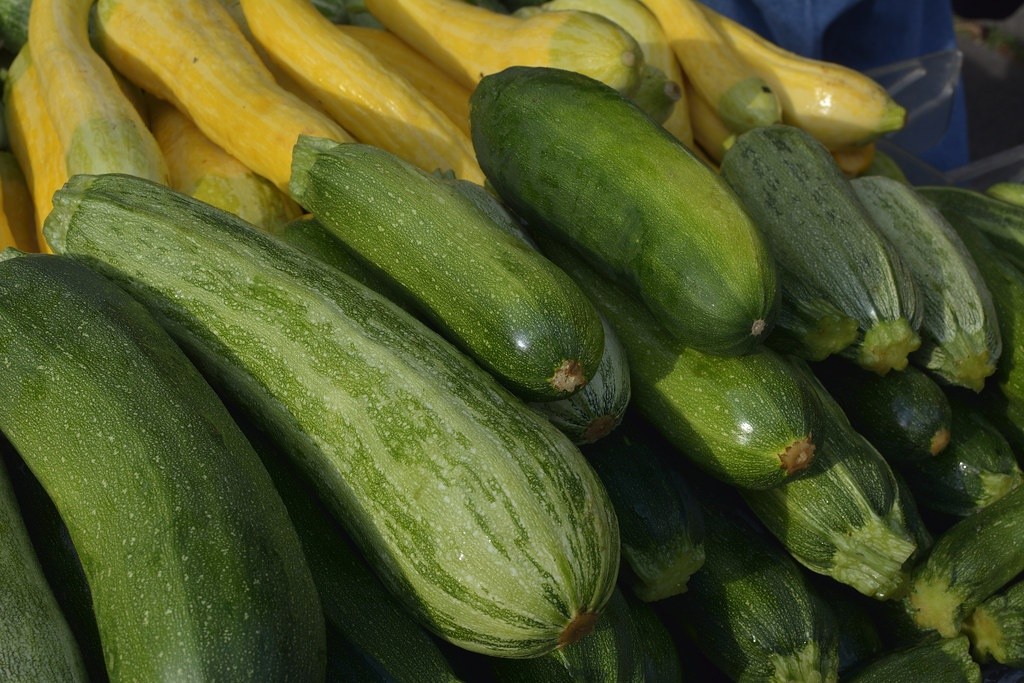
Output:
[0,0,1024,683]
